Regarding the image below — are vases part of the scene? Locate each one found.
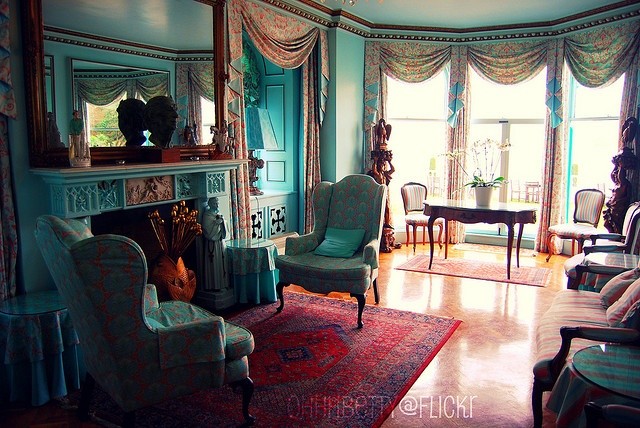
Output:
[475,186,495,207]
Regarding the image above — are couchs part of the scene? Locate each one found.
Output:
[28,212,259,427]
[563,201,640,288]
[530,263,640,427]
[273,172,390,329]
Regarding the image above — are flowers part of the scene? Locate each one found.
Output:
[447,134,512,190]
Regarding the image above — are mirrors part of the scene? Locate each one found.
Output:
[18,0,226,170]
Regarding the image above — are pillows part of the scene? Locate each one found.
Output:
[312,226,366,259]
[597,266,640,311]
[604,278,640,328]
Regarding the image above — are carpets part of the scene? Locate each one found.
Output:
[84,290,464,428]
[393,252,551,287]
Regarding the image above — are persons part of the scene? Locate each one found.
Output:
[69,109,85,158]
[144,94,181,148]
[202,196,229,290]
[117,99,148,147]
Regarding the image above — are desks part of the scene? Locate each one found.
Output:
[577,250,639,291]
[522,180,543,203]
[543,343,640,428]
[222,235,279,306]
[421,197,539,281]
[1,290,82,406]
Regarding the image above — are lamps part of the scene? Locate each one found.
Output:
[243,106,280,197]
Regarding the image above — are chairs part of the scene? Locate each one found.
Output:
[400,181,444,254]
[545,187,605,263]
[508,175,526,203]
